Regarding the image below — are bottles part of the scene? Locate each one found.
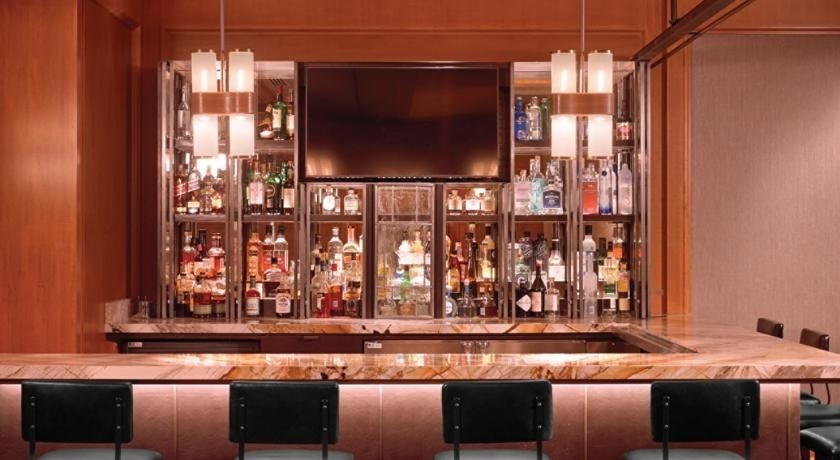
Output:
[446,189,497,212]
[445,222,497,316]
[173,229,226,317]
[572,224,631,319]
[262,83,294,141]
[582,161,632,215]
[614,99,633,146]
[514,95,552,142]
[244,154,296,214]
[513,155,563,214]
[310,224,361,317]
[175,163,226,214]
[178,85,190,138]
[378,230,432,318]
[309,183,360,215]
[506,231,566,318]
[243,224,294,319]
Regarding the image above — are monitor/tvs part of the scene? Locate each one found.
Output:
[290,60,513,186]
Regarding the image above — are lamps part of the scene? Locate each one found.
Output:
[185,0,262,164]
[546,0,618,166]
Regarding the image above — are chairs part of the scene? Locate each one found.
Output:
[621,371,762,460]
[226,376,357,460]
[433,377,555,460]
[756,312,786,340]
[794,325,840,460]
[16,378,166,460]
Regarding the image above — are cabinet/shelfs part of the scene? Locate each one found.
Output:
[361,339,586,356]
[125,338,261,355]
[158,53,654,326]
